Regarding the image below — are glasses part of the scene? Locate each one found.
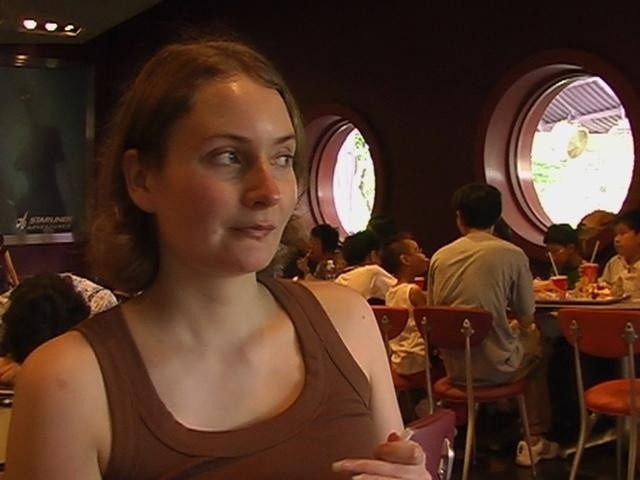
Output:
[544,248,566,258]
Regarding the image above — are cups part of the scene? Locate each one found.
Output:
[414,277,425,289]
[551,276,567,298]
[582,263,597,285]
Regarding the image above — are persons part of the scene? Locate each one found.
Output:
[0,35,432,479]
[424,184,568,466]
[0,270,118,391]
[297,222,440,417]
[543,210,640,443]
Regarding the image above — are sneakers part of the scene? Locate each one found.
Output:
[514,435,559,466]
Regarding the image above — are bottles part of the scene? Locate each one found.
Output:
[325,260,336,280]
